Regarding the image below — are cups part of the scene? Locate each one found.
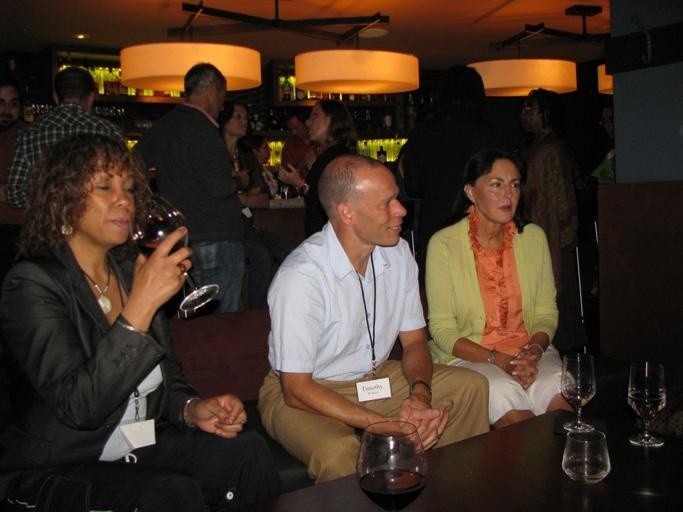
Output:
[355,420,430,512]
[561,430,612,512]
[125,194,220,315]
[560,353,596,434]
[627,362,667,448]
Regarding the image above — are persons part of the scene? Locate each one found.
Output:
[0,74,35,279]
[519,87,578,353]
[260,155,490,485]
[425,153,577,431]
[243,129,276,195]
[8,69,127,216]
[425,64,500,234]
[276,100,360,236]
[217,98,271,312]
[0,135,277,512]
[128,62,251,315]
[282,106,311,144]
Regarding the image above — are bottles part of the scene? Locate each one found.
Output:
[418,98,425,130]
[396,139,407,146]
[395,142,402,159]
[383,99,392,138]
[295,87,305,102]
[358,140,379,160]
[23,86,34,124]
[393,95,405,139]
[407,94,417,137]
[363,95,372,138]
[384,139,395,162]
[350,94,360,131]
[278,76,295,102]
[425,97,437,130]
[57,66,128,95]
[379,139,383,146]
[249,114,264,132]
[268,109,281,131]
[265,142,284,165]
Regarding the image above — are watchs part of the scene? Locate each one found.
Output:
[409,379,433,401]
[296,177,312,196]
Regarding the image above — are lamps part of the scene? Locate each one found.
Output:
[468,59,577,96]
[597,63,614,94]
[295,49,418,94]
[117,43,261,91]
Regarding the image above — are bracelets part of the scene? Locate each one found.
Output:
[184,394,199,430]
[489,347,496,366]
[524,342,547,356]
[116,320,150,335]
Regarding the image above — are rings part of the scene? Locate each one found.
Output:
[240,419,247,426]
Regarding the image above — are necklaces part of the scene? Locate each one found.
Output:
[81,267,114,314]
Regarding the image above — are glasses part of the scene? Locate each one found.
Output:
[523,104,549,115]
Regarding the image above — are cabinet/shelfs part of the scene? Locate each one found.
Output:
[14,44,264,137]
[264,61,476,136]
[238,191,313,298]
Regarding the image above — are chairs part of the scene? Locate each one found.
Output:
[166,305,277,405]
